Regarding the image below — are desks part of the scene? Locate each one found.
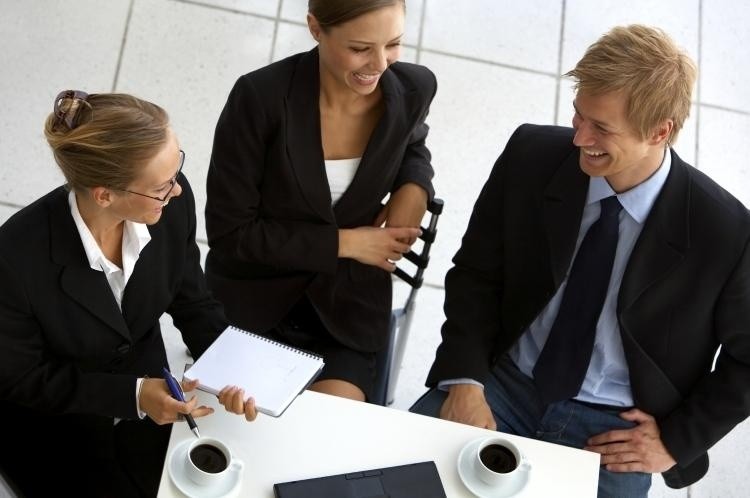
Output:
[153,380,602,497]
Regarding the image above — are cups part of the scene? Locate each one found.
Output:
[183,438,244,486]
[475,436,532,486]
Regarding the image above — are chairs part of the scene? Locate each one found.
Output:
[386,193,445,404]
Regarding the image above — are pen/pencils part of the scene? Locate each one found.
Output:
[163,366,201,439]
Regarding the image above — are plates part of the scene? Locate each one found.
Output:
[457,438,530,498]
[168,437,241,498]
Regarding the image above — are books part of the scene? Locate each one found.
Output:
[183,324,325,417]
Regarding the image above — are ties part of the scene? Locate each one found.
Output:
[532,195,625,400]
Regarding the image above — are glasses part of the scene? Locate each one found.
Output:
[123,149,185,201]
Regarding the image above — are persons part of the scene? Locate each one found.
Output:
[411,24,750,498]
[1,90,257,498]
[200,0,439,402]
